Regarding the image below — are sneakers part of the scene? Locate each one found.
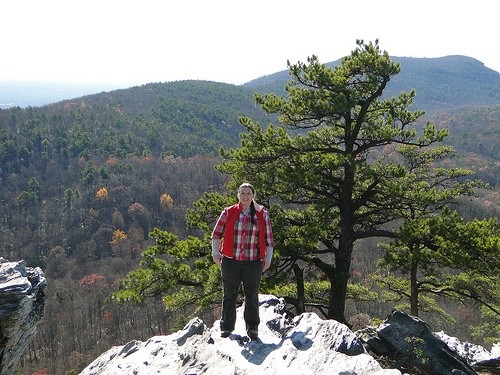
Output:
[246,322,259,338]
[221,329,231,338]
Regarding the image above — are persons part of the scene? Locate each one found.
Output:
[211,182,275,339]
